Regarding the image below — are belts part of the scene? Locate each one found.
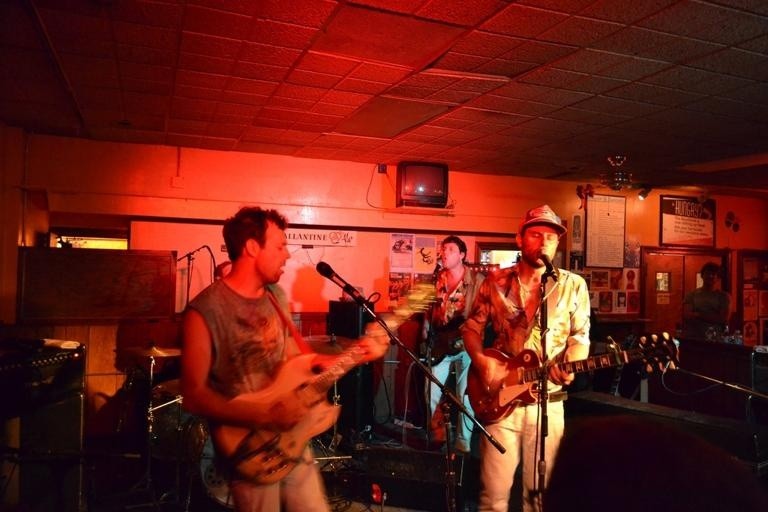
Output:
[539,389,569,401]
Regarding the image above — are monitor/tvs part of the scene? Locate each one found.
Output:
[396,161,448,208]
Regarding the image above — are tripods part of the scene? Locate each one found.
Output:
[129,359,191,512]
[320,382,352,496]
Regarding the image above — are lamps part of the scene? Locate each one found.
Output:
[573,181,652,201]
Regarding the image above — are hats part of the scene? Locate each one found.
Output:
[518,204,568,237]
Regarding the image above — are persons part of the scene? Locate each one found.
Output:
[177,204,391,511]
[393,241,400,249]
[457,202,593,512]
[680,261,730,327]
[417,234,484,457]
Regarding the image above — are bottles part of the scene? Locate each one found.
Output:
[704,324,743,345]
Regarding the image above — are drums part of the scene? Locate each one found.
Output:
[205,457,236,510]
[150,406,207,464]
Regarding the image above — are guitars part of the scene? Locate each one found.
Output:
[216,284,438,485]
[415,314,492,366]
[467,332,680,423]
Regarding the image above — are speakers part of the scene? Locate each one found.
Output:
[0,393,85,512]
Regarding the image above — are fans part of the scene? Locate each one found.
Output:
[597,152,633,195]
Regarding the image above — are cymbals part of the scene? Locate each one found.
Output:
[122,346,183,357]
[151,376,194,397]
[298,335,357,355]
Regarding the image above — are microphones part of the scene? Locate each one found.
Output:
[316,261,366,306]
[538,249,558,282]
[434,260,442,274]
